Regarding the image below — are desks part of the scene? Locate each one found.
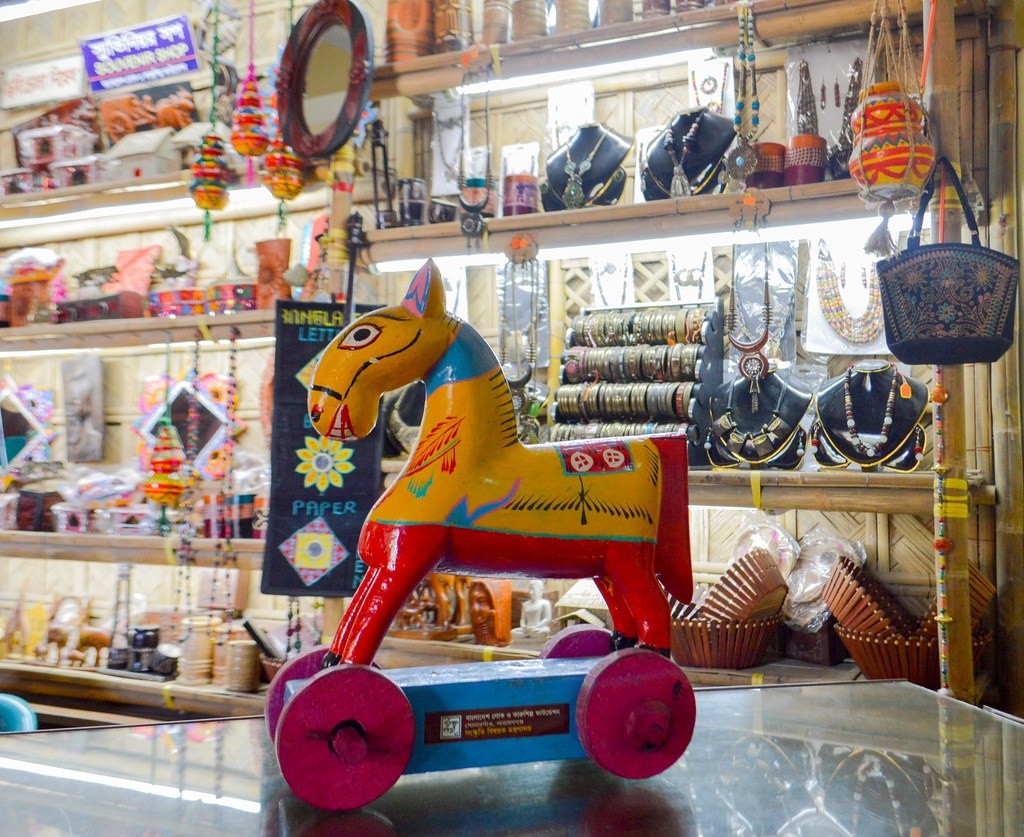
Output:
[0,677,1024,837]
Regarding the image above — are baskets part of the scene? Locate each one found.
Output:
[823,557,920,638]
[667,616,785,671]
[833,622,993,691]
[698,546,789,625]
[913,557,997,640]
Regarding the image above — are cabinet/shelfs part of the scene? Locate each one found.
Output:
[0,0,999,727]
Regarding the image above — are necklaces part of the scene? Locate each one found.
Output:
[343,0,934,416]
[109,328,324,670]
[680,746,908,837]
[845,360,897,456]
[382,386,421,454]
[713,369,790,458]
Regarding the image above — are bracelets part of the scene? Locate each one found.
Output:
[550,308,706,442]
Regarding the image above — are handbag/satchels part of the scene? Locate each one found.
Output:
[874,155,1021,366]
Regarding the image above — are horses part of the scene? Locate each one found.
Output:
[309,257,693,660]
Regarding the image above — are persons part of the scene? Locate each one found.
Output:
[468,579,512,646]
[510,580,552,642]
